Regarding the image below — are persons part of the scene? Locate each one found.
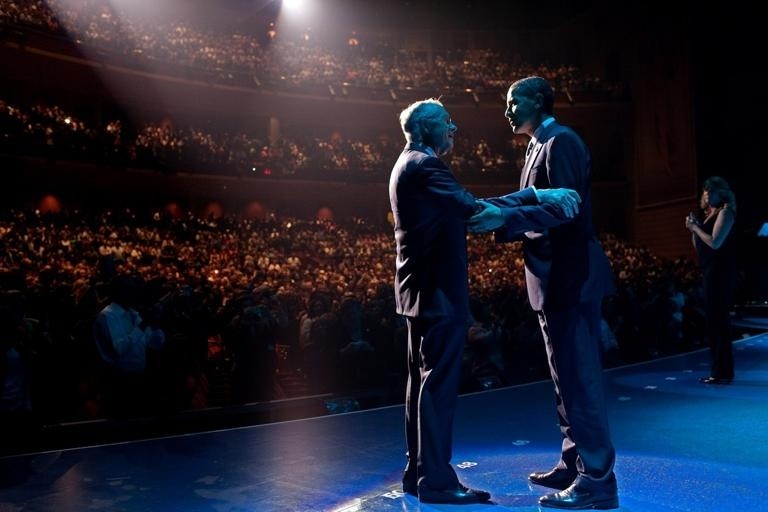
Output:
[685,176,738,385]
[388,97,584,506]
[464,74,621,510]
[0,1,705,414]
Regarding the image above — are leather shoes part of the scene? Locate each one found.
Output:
[404,474,490,502]
[698,377,733,385]
[538,484,619,510]
[530,469,573,488]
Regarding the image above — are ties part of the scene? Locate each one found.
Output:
[526,143,533,156]
